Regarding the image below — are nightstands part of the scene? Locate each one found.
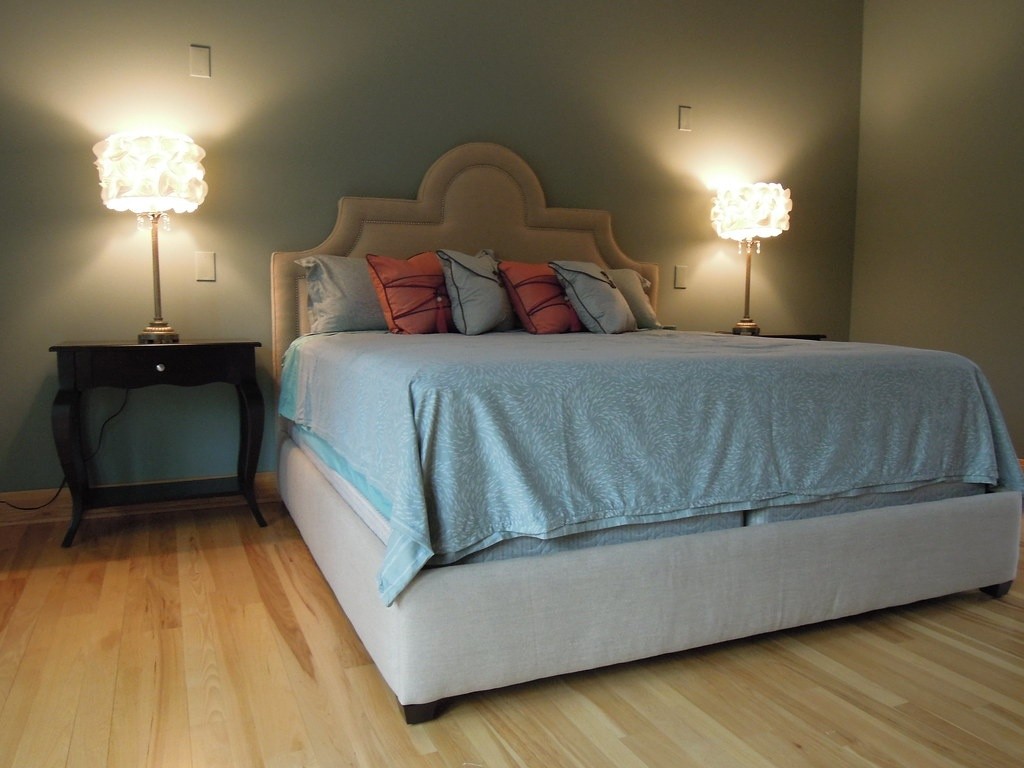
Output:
[758,334,827,341]
[51,339,268,548]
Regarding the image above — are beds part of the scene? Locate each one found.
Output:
[265,141,1024,725]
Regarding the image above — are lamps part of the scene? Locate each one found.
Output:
[89,120,208,347]
[708,180,792,337]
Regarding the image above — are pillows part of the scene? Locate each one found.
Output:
[548,261,640,334]
[432,245,515,336]
[365,251,455,336]
[292,254,389,335]
[496,259,585,335]
[609,268,663,329]
